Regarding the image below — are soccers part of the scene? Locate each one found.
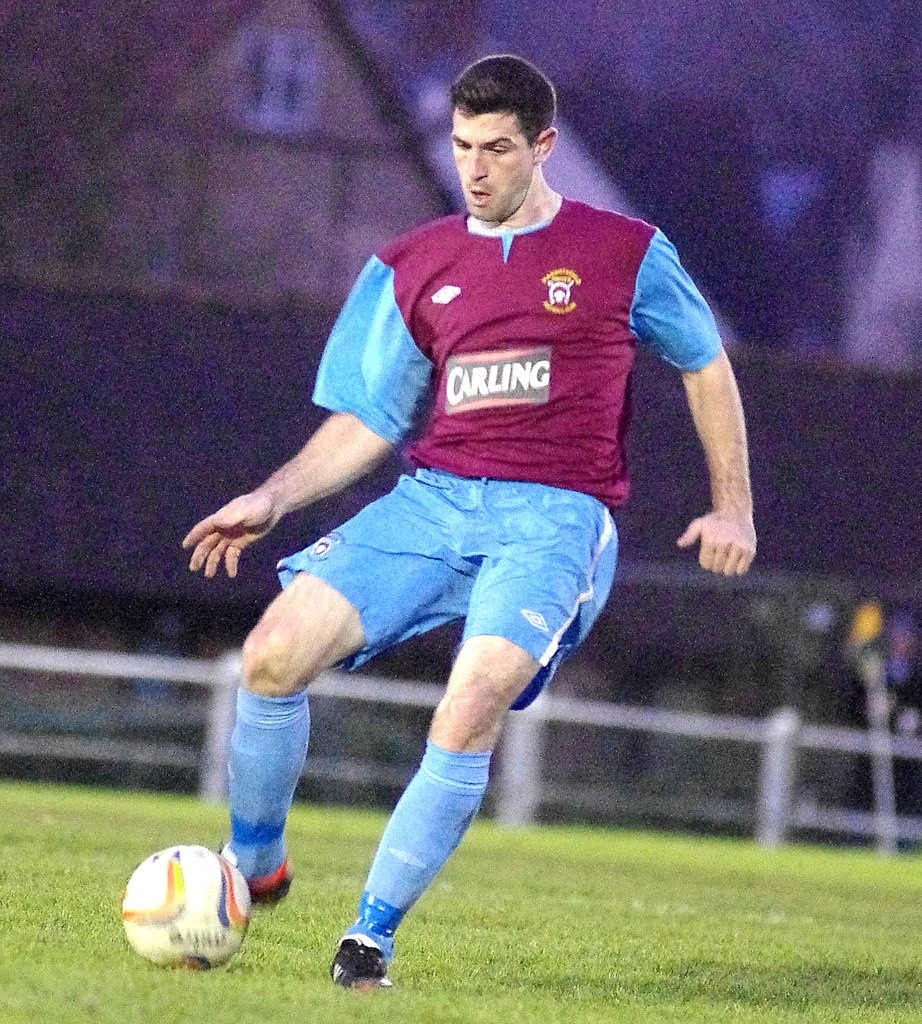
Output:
[121,844,251,971]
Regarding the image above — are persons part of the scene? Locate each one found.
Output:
[179,54,757,991]
[835,606,922,820]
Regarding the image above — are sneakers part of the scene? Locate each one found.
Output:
[220,838,294,903]
[332,935,388,988]
